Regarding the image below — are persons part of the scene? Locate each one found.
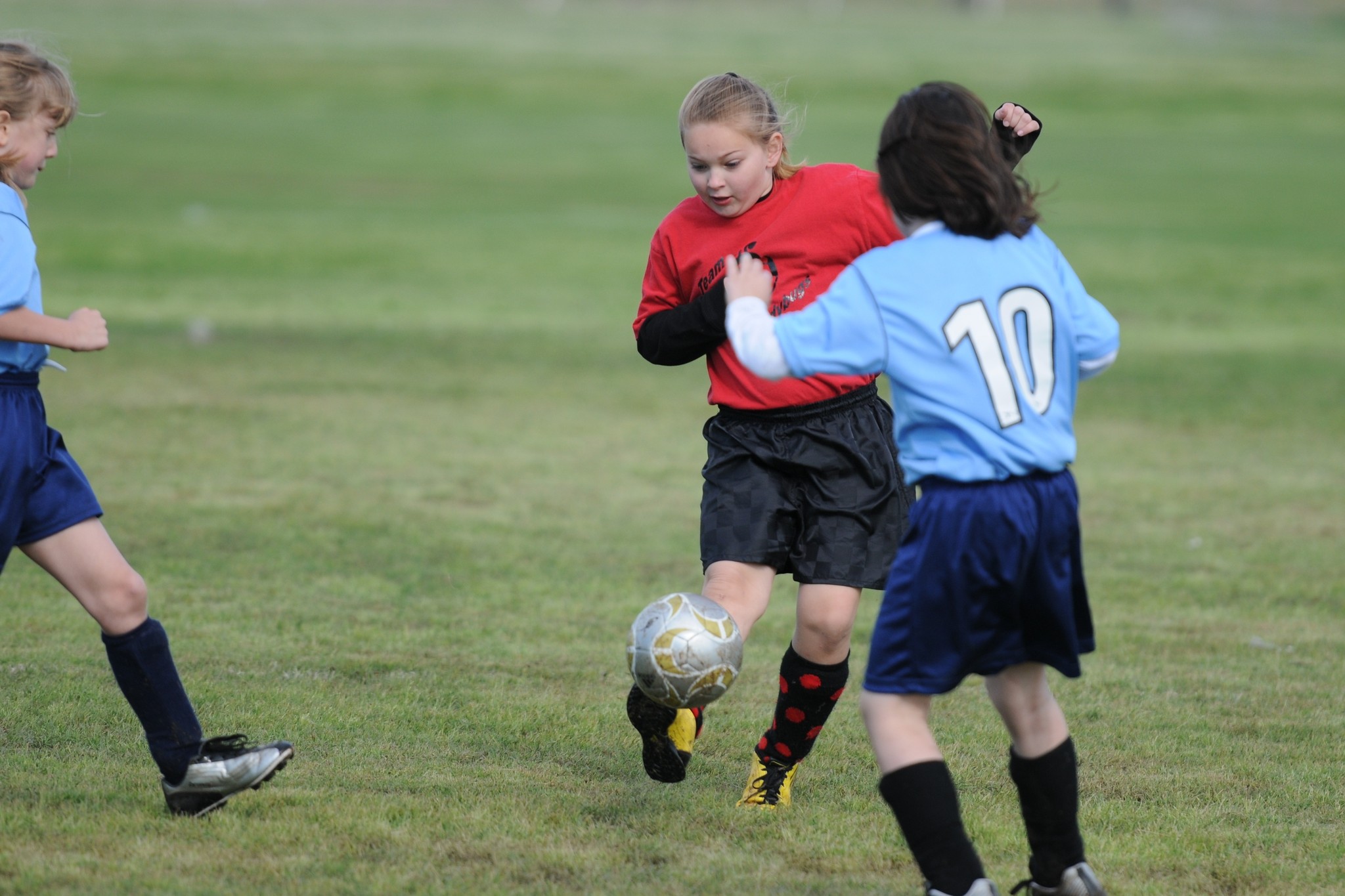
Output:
[0,39,295,818]
[627,75,1047,811]
[720,80,1122,896]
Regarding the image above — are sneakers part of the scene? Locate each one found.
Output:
[160,738,295,821]
[734,743,799,811]
[626,682,698,783]
[1026,861,1107,896]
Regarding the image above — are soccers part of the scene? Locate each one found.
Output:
[627,591,743,708]
